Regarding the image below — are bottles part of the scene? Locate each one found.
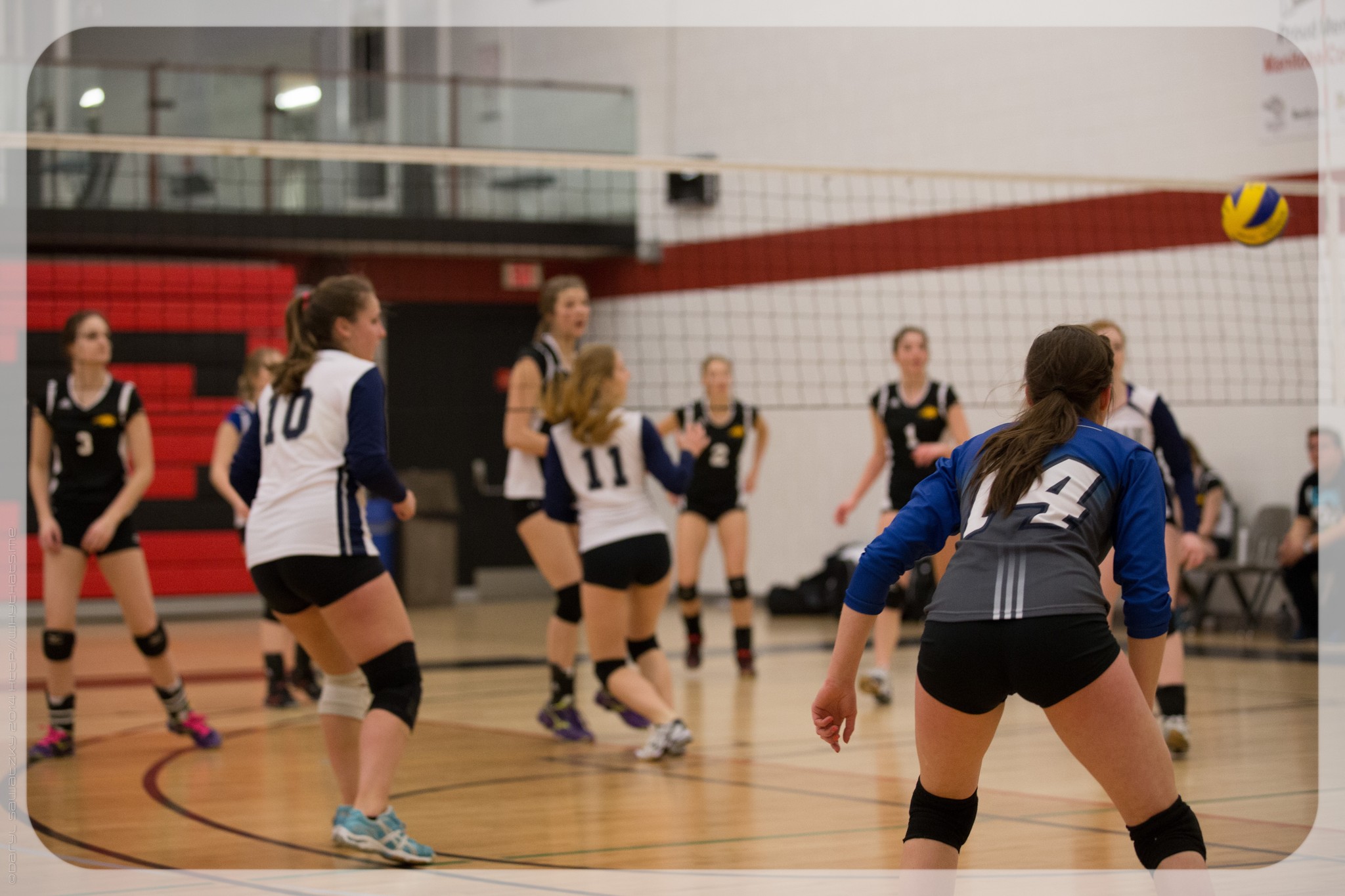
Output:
[1237,523,1249,565]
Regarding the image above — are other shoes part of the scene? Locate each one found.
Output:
[736,647,754,676]
[291,667,321,696]
[1157,714,1191,761]
[538,704,592,744]
[267,678,292,706]
[686,640,700,668]
[1288,625,1318,642]
[634,717,693,761]
[595,686,649,728]
[858,667,894,705]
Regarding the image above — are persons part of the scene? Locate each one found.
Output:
[212,272,1345,875]
[811,321,1214,896]
[25,311,222,758]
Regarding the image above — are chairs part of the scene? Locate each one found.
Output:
[1176,501,1296,631]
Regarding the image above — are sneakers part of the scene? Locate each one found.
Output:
[167,709,222,747]
[331,805,437,864]
[27,726,75,760]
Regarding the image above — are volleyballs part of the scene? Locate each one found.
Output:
[1219,180,1290,248]
